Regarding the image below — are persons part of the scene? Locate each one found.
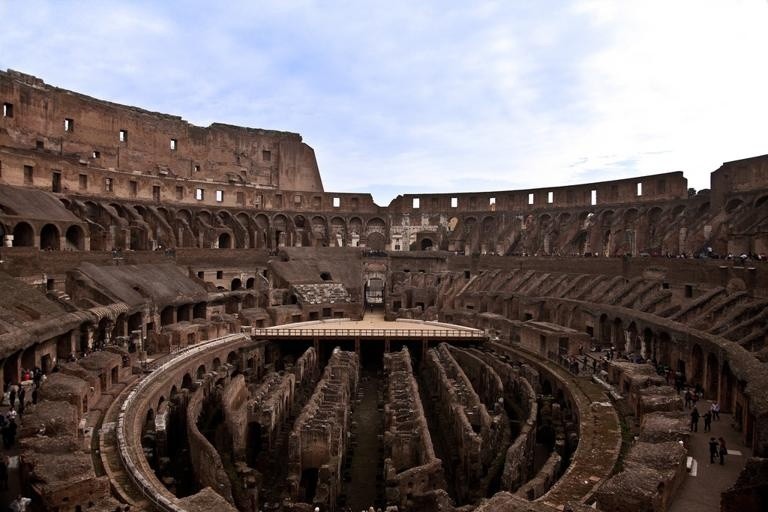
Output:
[476,247,768,263]
[561,340,728,467]
[0,334,136,508]
[38,243,164,251]
[377,294,380,300]
[370,303,374,312]
[256,497,400,512]
